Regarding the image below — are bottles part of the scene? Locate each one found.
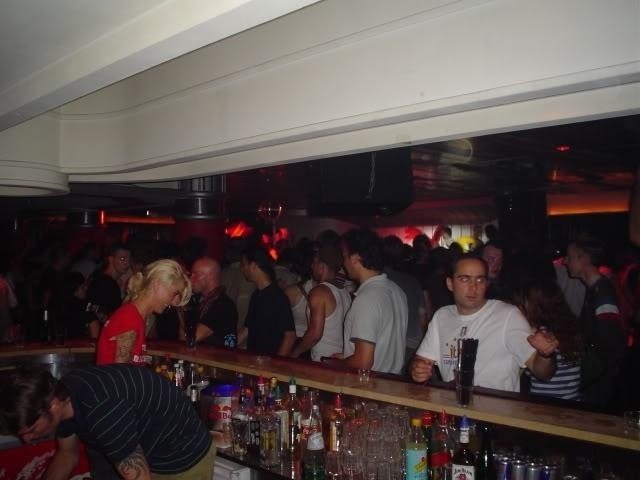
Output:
[301,405,326,480]
[404,411,495,480]
[161,352,322,468]
[39,304,53,345]
[329,393,345,454]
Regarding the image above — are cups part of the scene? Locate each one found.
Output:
[55,328,67,347]
[623,410,640,438]
[453,367,474,408]
[327,398,411,480]
[15,326,26,346]
[184,335,196,351]
[223,334,236,350]
[358,368,375,386]
